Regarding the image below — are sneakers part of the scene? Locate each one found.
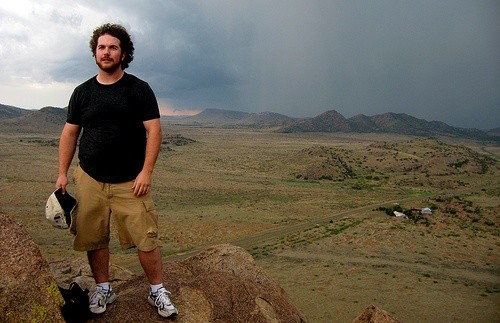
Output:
[148,286,179,318]
[89,283,117,313]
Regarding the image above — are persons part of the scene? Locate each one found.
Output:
[55,23,179,319]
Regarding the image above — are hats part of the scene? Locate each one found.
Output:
[45,186,78,231]
